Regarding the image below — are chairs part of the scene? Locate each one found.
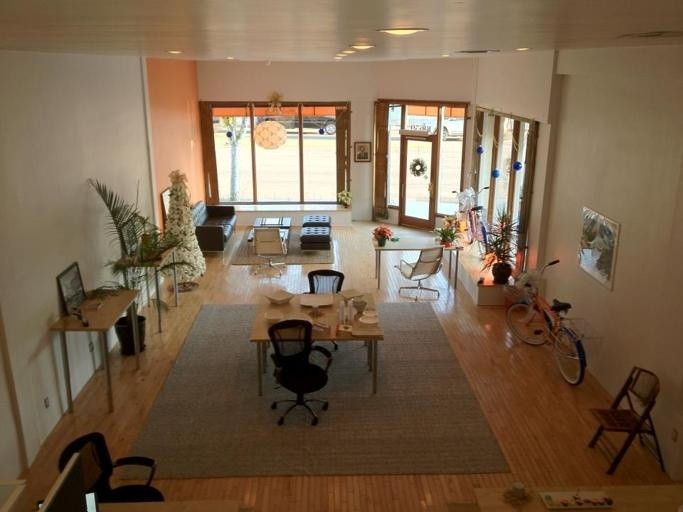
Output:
[56,431,164,502]
[393,245,445,301]
[267,319,332,427]
[307,269,344,293]
[254,228,286,276]
[586,366,668,475]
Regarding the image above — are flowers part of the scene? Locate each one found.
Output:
[372,225,393,241]
[408,158,427,177]
[337,190,351,205]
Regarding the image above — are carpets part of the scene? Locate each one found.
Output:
[230,226,335,267]
[117,299,511,481]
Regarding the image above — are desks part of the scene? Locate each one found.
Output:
[48,288,141,415]
[247,228,290,251]
[371,237,463,289]
[473,484,683,511]
[95,500,240,511]
[117,239,180,333]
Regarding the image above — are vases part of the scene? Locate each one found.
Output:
[377,238,386,247]
[341,202,348,209]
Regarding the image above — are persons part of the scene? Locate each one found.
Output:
[356,146,368,160]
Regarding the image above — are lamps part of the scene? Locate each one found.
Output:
[250,118,287,151]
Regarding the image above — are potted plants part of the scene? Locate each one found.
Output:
[83,177,195,355]
[437,228,456,247]
[479,210,521,285]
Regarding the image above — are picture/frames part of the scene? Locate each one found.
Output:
[575,205,621,292]
[353,141,371,162]
[56,263,85,312]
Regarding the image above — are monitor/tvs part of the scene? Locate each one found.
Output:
[37,491,99,512]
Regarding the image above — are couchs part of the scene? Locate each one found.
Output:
[189,200,236,251]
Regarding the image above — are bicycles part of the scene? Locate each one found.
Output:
[506,257,586,387]
[452,185,491,260]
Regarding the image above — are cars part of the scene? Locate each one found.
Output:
[245,114,336,136]
[428,118,464,139]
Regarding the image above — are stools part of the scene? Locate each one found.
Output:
[302,215,331,233]
[299,227,331,251]
